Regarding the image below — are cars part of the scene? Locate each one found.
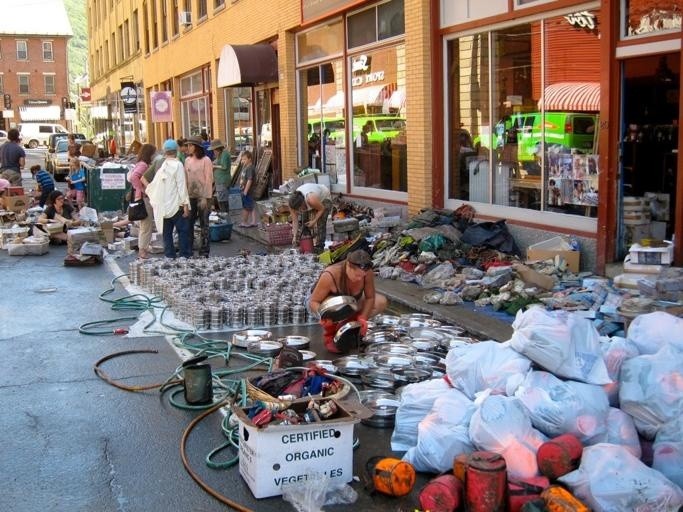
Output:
[0,130,9,145]
[50,139,94,182]
[43,134,85,171]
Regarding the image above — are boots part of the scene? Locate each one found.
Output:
[323,321,363,354]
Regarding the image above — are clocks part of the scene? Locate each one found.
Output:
[119,81,138,113]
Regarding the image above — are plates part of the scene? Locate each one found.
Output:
[232,328,273,350]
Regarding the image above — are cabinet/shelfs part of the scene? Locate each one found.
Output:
[81,157,133,214]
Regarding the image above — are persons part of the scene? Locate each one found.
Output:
[184,136,214,259]
[239,150,259,229]
[140,152,181,187]
[113,190,139,251]
[287,182,333,256]
[126,144,157,259]
[353,124,371,188]
[108,135,116,159]
[176,138,189,167]
[307,249,388,354]
[547,179,560,205]
[200,131,214,162]
[66,133,83,163]
[64,157,86,213]
[207,138,232,243]
[17,135,23,146]
[37,190,82,246]
[0,128,27,187]
[30,165,55,208]
[145,138,192,261]
[573,179,585,202]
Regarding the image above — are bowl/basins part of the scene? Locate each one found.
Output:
[278,336,313,350]
[284,348,317,361]
[302,360,339,375]
[129,247,325,331]
[331,311,481,428]
[249,340,284,357]
[320,294,359,318]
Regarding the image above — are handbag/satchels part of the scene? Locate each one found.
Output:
[299,226,315,253]
[127,185,148,221]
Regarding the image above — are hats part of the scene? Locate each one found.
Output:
[184,135,205,149]
[207,138,226,152]
[163,138,179,152]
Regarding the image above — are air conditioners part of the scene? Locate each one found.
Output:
[179,11,193,26]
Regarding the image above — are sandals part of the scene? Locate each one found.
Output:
[237,221,259,229]
[139,249,149,260]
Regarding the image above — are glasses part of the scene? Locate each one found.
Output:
[350,260,374,272]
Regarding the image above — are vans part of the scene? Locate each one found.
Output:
[472,112,599,173]
[352,115,406,146]
[307,117,345,142]
[17,122,72,149]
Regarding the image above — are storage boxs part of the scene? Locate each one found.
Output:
[43,223,66,235]
[208,223,232,243]
[0,195,31,214]
[374,205,410,228]
[232,397,376,501]
[333,217,360,233]
[227,194,244,210]
[5,237,52,257]
[256,224,294,246]
[101,222,116,244]
[528,247,580,275]
[331,233,350,241]
[229,188,240,194]
[318,175,331,195]
[621,196,646,212]
[629,241,674,265]
[647,190,671,221]
[632,223,667,244]
[301,174,317,187]
[622,212,651,227]
[1,227,30,251]
